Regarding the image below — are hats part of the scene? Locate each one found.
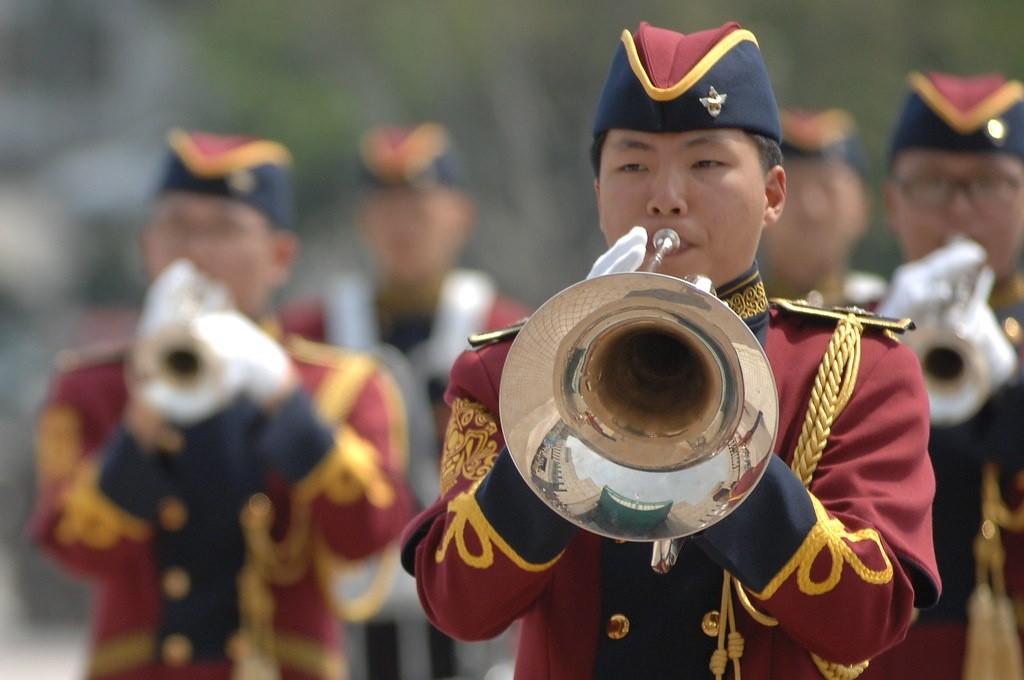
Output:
[160,126,293,229]
[356,123,468,194]
[775,103,861,167]
[888,72,1024,170]
[590,25,781,176]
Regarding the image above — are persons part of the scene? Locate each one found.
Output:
[713,458,767,503]
[283,122,535,680]
[732,410,763,452]
[584,410,617,442]
[868,74,1024,680]
[401,22,944,680]
[754,109,887,313]
[38,131,414,680]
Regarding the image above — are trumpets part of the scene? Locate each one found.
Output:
[121,268,242,426]
[496,228,781,573]
[889,234,992,427]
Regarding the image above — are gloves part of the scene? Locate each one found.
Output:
[587,226,648,280]
[136,262,293,409]
[874,238,1017,395]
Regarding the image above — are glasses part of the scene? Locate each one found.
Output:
[891,173,1024,214]
